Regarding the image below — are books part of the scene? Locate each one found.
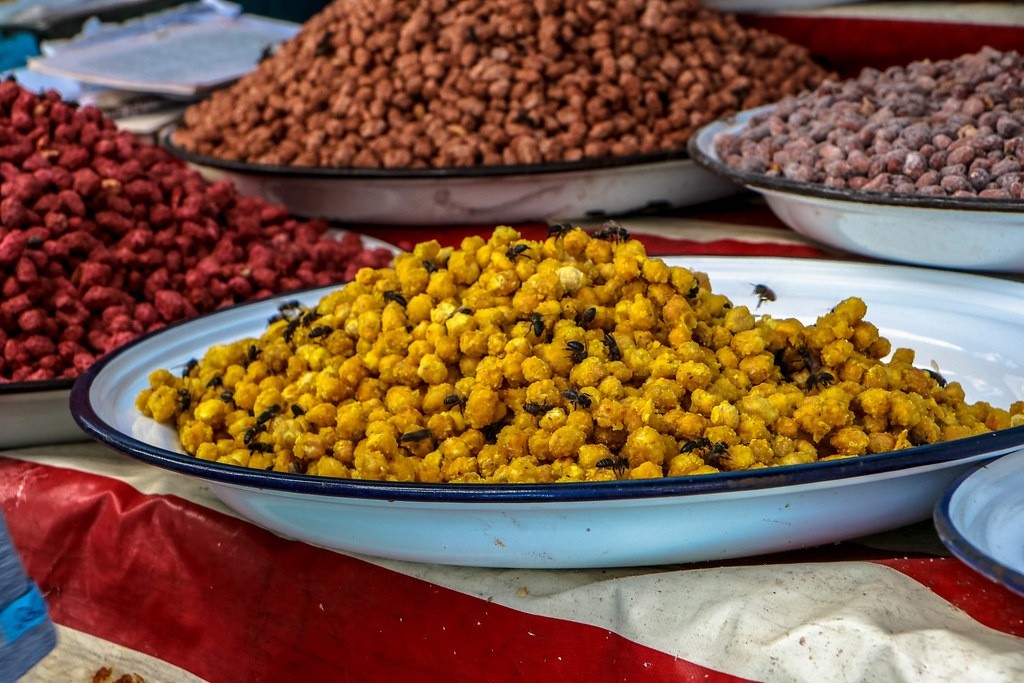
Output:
[27,11,308,96]
